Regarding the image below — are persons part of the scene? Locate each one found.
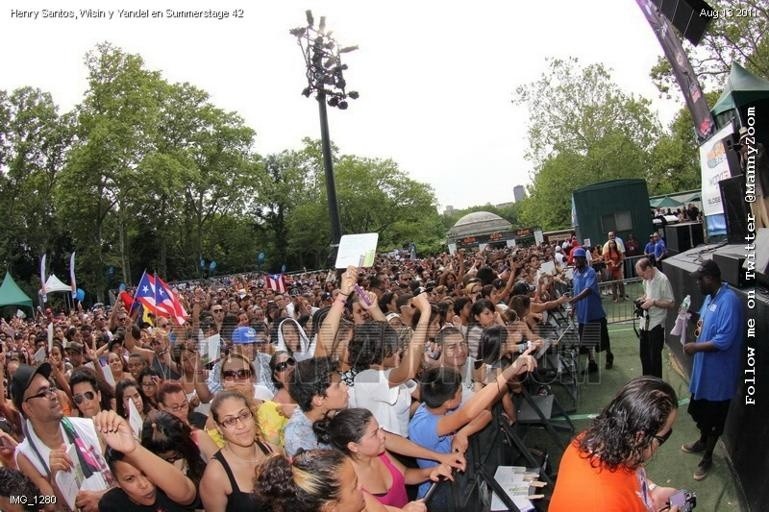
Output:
[654,232,666,272]
[738,126,769,246]
[0,230,641,512]
[644,233,656,267]
[681,258,745,481]
[635,258,676,380]
[656,202,703,222]
[548,375,679,512]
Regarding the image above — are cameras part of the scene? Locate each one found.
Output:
[633,298,648,317]
[668,487,697,511]
[732,143,743,150]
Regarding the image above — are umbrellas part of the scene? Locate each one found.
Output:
[649,193,702,208]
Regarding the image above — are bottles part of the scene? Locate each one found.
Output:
[682,295,691,311]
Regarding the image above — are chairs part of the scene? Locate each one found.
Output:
[424,282,582,511]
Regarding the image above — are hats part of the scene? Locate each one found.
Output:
[555,246,562,252]
[10,362,52,400]
[231,326,265,345]
[413,286,433,296]
[688,259,721,279]
[289,288,301,298]
[572,248,586,258]
[321,292,334,302]
[513,280,536,295]
[64,341,84,353]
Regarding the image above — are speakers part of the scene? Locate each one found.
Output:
[721,131,745,177]
[718,174,755,244]
[664,222,704,253]
[712,252,753,289]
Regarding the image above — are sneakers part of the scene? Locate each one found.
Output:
[605,354,614,369]
[680,439,706,453]
[586,361,599,374]
[693,452,713,480]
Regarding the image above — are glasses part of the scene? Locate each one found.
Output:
[694,320,704,338]
[141,381,155,387]
[217,410,254,428]
[22,388,55,402]
[401,277,413,282]
[271,357,296,372]
[210,308,225,314]
[220,368,251,381]
[647,429,673,446]
